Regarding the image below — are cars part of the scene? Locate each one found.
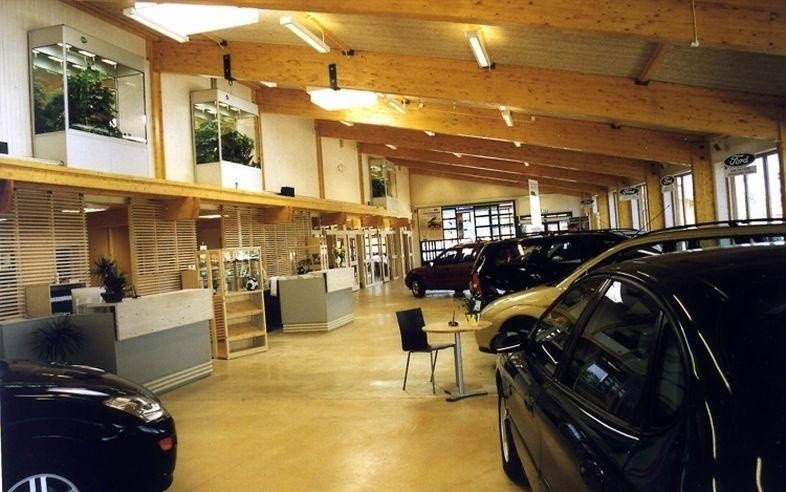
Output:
[0,353,177,492]
[489,245,786,491]
[548,228,662,258]
[405,240,498,297]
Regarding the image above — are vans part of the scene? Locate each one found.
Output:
[473,217,786,358]
[468,228,664,313]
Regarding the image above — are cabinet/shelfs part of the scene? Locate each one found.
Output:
[181,268,200,289]
[196,245,269,360]
[24,280,86,319]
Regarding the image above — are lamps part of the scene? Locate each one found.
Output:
[463,29,491,67]
[279,15,330,54]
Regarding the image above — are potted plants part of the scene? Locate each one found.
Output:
[88,255,127,302]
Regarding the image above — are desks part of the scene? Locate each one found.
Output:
[419,320,493,400]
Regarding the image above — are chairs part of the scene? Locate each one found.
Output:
[396,305,455,394]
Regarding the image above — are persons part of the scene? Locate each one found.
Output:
[568,223,579,231]
